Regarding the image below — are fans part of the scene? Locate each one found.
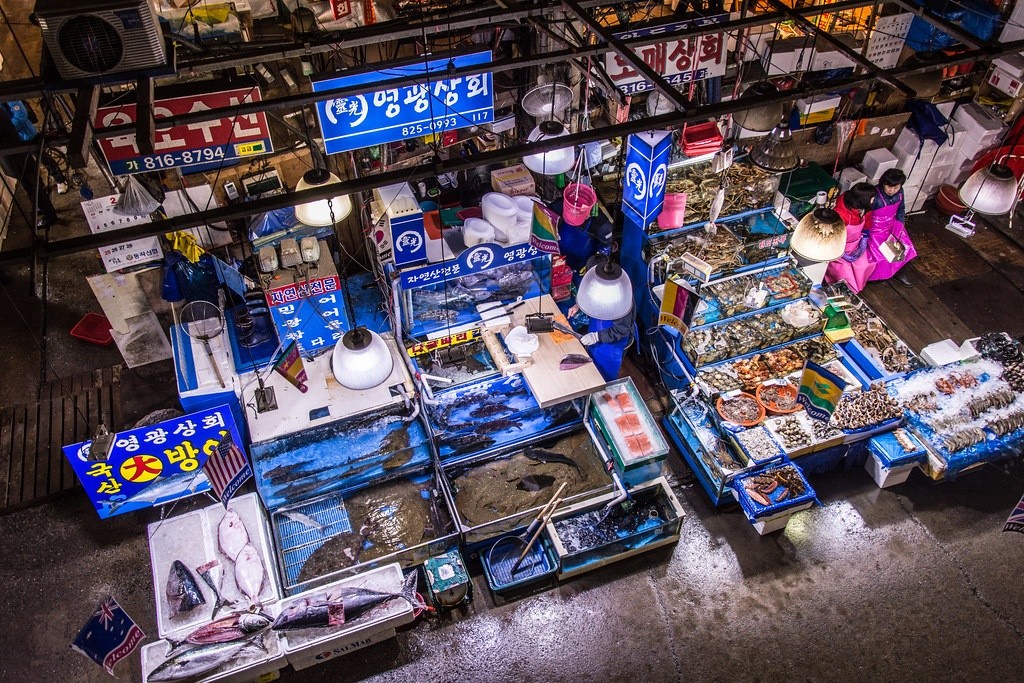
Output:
[522,73,574,120]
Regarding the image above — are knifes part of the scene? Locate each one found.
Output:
[477,311,515,324]
[505,300,524,313]
[473,301,511,316]
[552,321,584,340]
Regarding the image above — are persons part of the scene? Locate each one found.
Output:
[821,184,879,297]
[567,299,638,384]
[550,190,615,293]
[8,101,70,197]
[0,114,58,230]
[867,167,919,292]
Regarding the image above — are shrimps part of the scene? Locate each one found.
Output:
[904,369,1024,454]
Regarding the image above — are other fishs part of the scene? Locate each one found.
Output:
[262,458,340,500]
[411,263,536,322]
[217,508,264,612]
[196,558,237,621]
[165,559,207,621]
[279,477,456,585]
[584,508,676,557]
[430,390,573,453]
[146,612,271,683]
[270,567,430,632]
[523,448,584,479]
[377,421,414,470]
[337,450,384,480]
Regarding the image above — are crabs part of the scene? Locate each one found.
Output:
[702,438,743,481]
[850,310,917,373]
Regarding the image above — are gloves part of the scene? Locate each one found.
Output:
[842,254,857,262]
[851,238,867,260]
[579,332,599,346]
[892,221,903,239]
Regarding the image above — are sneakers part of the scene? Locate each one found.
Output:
[31,207,42,217]
[37,215,59,229]
[57,179,69,194]
[892,270,913,287]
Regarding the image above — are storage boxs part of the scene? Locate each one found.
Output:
[864,456,911,489]
[917,332,1016,368]
[832,100,1009,214]
[748,514,791,537]
[138,312,690,682]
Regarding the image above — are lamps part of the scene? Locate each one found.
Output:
[958,164,1019,216]
[749,118,802,174]
[573,263,634,319]
[731,80,784,133]
[522,121,577,178]
[297,168,353,227]
[896,54,944,101]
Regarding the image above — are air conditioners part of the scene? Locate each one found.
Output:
[32,0,168,79]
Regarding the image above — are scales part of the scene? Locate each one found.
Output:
[652,252,720,328]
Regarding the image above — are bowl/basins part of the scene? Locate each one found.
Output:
[937,184,967,215]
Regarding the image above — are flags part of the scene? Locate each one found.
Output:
[1000,493,1024,536]
[794,360,847,426]
[203,429,254,513]
[656,278,701,342]
[273,340,309,394]
[69,597,145,679]
[527,203,563,256]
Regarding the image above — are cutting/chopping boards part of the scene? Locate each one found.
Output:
[503,293,607,410]
